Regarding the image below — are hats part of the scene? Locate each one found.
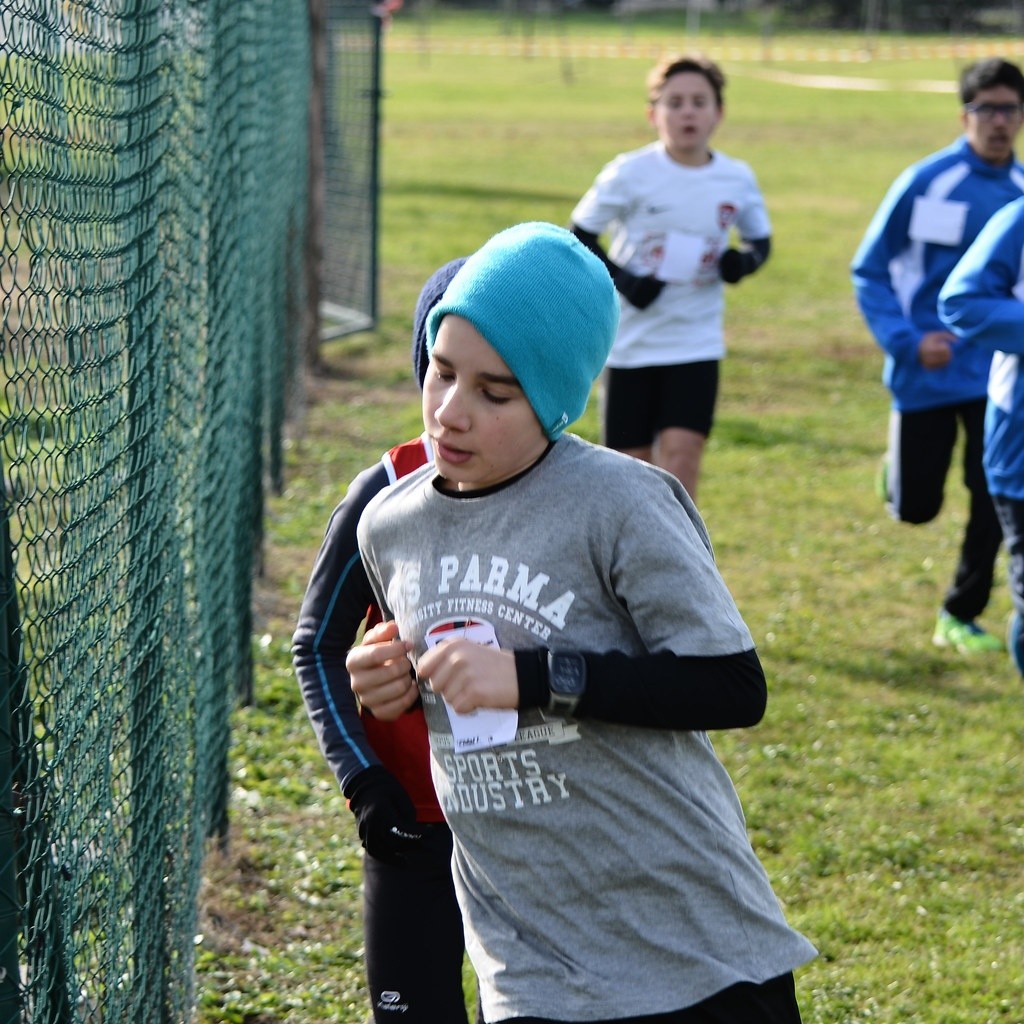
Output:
[414,221,620,442]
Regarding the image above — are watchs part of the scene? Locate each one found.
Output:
[539,640,586,719]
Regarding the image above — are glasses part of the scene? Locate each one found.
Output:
[963,99,1023,124]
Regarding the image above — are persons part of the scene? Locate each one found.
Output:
[851,56,1024,658]
[568,53,773,508]
[291,256,597,1023]
[934,192,1024,676]
[347,219,821,1024]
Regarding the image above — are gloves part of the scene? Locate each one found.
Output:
[624,272,663,311]
[719,246,756,286]
[346,770,429,862]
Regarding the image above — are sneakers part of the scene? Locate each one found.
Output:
[876,451,893,510]
[932,609,1000,655]
